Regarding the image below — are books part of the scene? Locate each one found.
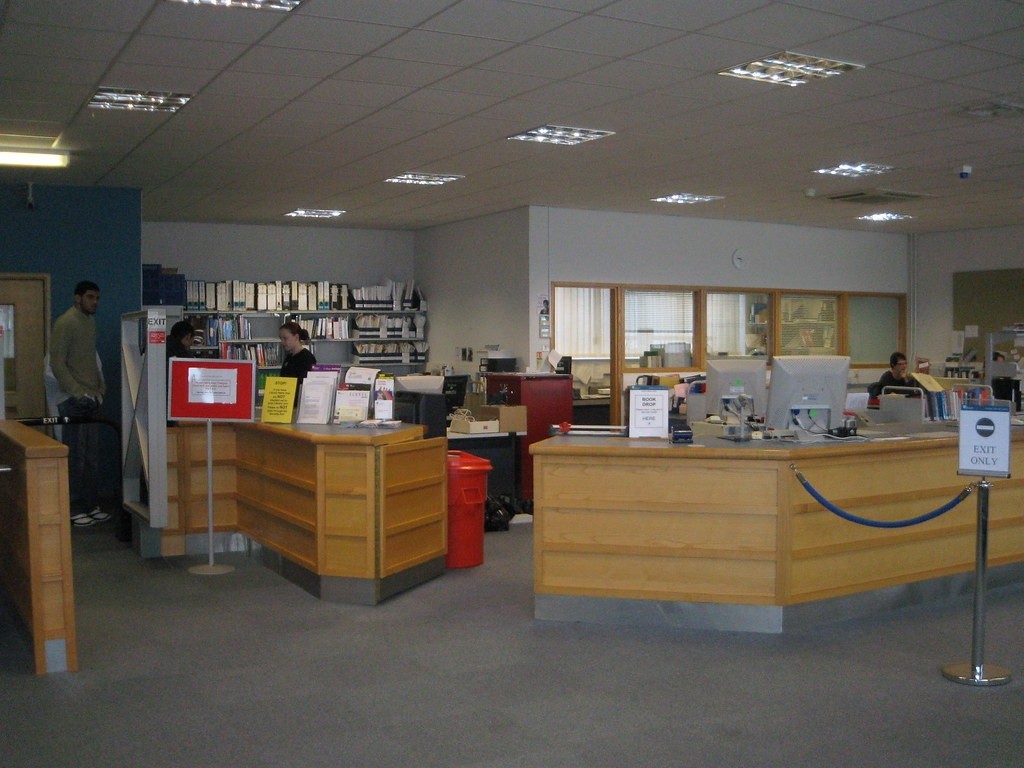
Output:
[925,389,989,421]
[350,280,429,365]
[186,279,349,389]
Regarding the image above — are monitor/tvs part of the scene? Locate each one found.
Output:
[555,356,572,374]
[393,375,469,411]
[706,356,850,442]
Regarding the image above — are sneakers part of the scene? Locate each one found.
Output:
[88,509,111,521]
[71,514,96,526]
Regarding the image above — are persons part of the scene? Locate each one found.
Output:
[992,351,1011,381]
[166,321,194,427]
[540,300,549,314]
[870,352,915,399]
[279,322,317,407]
[49,280,112,527]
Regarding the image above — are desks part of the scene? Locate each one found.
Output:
[446,429,528,518]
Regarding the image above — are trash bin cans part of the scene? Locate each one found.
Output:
[445,450,492,569]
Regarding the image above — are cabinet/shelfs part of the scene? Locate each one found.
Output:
[484,372,573,508]
[747,295,837,357]
[141,305,427,369]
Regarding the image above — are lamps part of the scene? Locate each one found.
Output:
[0,146,71,169]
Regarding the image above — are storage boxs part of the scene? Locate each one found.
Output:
[479,404,527,433]
